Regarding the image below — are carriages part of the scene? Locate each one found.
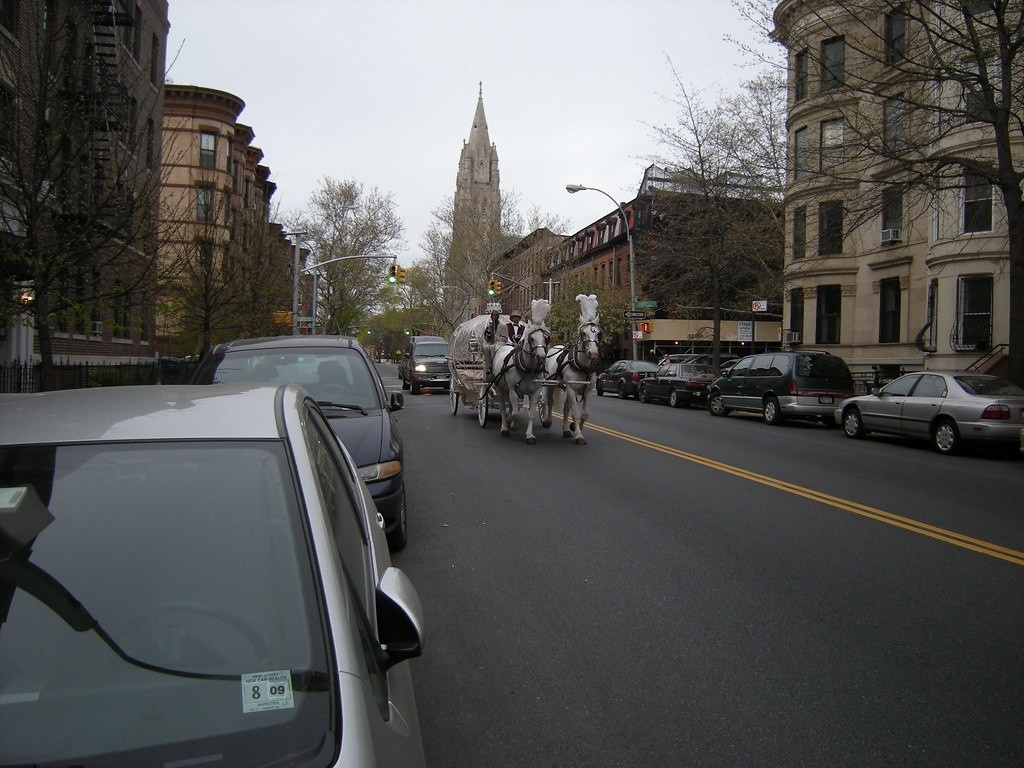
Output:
[449,293,603,446]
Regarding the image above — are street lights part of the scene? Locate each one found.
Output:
[311,204,359,334]
[399,267,405,283]
[389,265,396,283]
[566,184,639,361]
[439,284,469,321]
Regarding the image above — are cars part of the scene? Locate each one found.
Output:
[721,358,741,376]
[639,363,720,408]
[834,370,1024,456]
[596,359,660,399]
[0,381,433,768]
[398,359,402,379]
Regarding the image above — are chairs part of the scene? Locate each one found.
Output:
[315,360,348,392]
[254,361,278,382]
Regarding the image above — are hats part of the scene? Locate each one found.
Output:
[510,310,522,322]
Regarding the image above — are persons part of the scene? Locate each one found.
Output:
[483,311,508,382]
[506,310,526,346]
[247,363,279,384]
[318,360,356,399]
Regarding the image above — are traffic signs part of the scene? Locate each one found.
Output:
[624,311,646,319]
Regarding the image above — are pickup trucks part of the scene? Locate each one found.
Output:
[402,334,453,395]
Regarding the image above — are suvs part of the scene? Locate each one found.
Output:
[179,335,409,552]
[705,349,855,424]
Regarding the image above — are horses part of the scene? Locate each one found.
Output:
[492,314,603,446]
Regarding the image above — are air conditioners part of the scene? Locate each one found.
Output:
[0,156,14,172]
[881,227,900,243]
[42,180,57,198]
[786,332,801,342]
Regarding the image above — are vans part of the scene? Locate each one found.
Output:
[657,354,740,366]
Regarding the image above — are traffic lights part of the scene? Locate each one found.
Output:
[496,282,502,295]
[637,322,653,334]
[489,280,495,295]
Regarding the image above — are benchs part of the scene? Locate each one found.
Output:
[454,361,483,384]
